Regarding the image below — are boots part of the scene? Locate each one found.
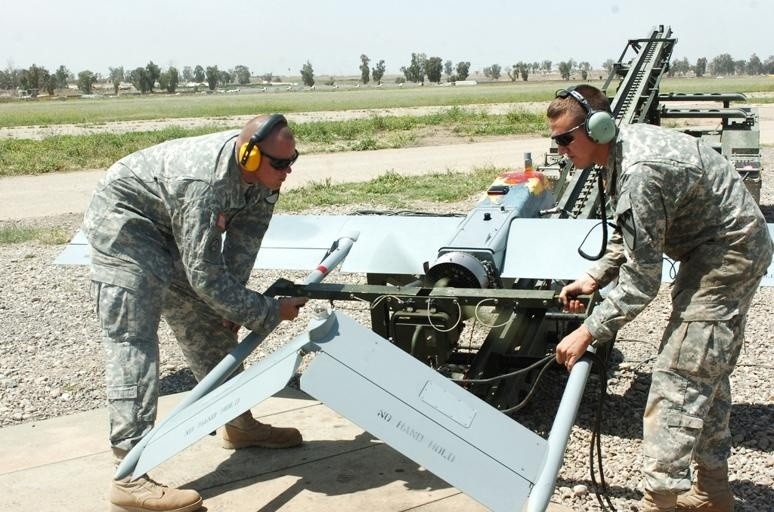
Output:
[630,491,677,511]
[109,448,203,511]
[221,411,303,449]
[678,470,734,512]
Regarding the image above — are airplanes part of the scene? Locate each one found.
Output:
[50,156,774,512]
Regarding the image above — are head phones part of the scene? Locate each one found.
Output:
[555,88,616,144]
[238,114,287,172]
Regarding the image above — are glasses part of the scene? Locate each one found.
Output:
[551,125,584,147]
[259,149,300,170]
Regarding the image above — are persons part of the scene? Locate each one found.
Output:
[85,114,307,512]
[547,85,773,512]
